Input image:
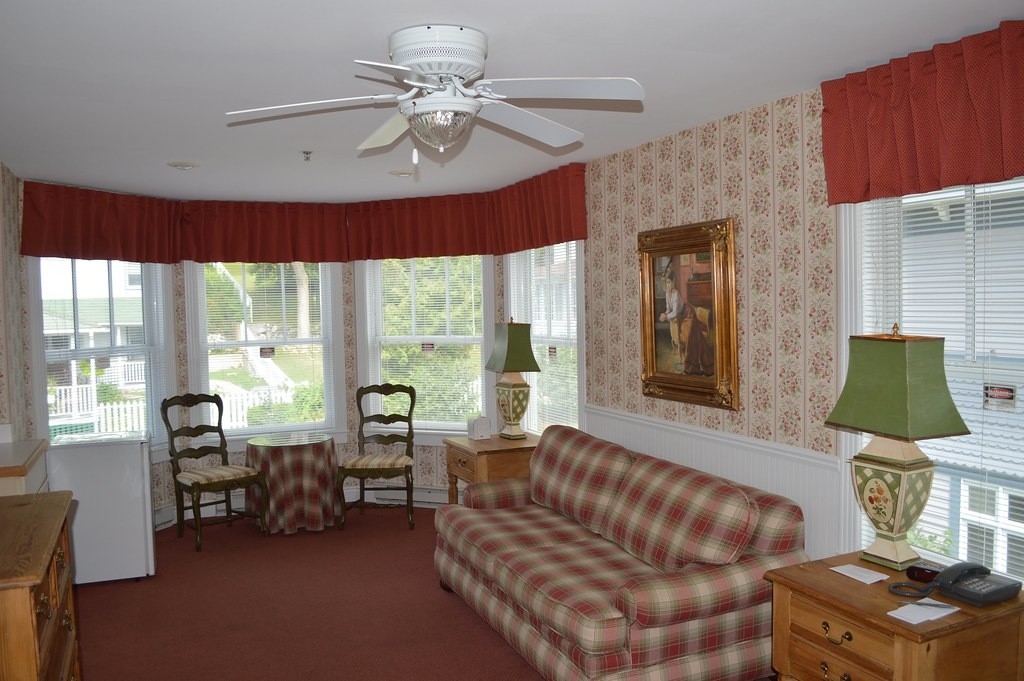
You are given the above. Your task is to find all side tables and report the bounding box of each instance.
[762,549,1024,681]
[443,431,541,506]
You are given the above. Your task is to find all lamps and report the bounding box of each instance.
[399,95,484,151]
[825,321,960,568]
[484,317,541,441]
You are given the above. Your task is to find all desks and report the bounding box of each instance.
[243,432,344,535]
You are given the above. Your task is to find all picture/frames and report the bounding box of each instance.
[634,218,738,410]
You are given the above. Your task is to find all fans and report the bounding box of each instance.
[220,25,647,165]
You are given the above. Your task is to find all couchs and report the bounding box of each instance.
[434,423,803,681]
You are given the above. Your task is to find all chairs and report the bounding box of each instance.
[335,384,419,531]
[159,393,268,552]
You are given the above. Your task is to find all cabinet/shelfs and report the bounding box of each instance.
[47,433,156,585]
[0,489,87,681]
[0,439,50,498]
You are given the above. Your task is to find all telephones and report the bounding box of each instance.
[932,561,1021,607]
